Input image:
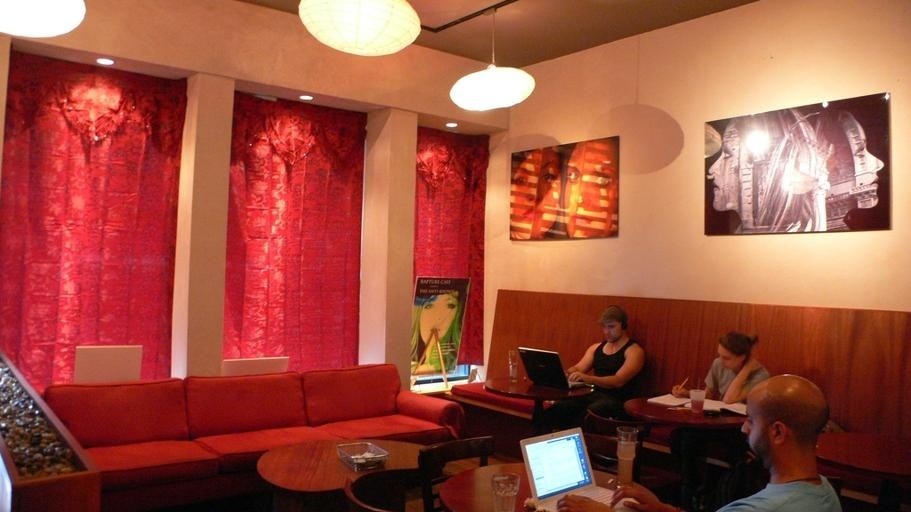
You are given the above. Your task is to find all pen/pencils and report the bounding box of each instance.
[678,376,689,390]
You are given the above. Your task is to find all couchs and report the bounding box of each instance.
[36,362,468,512]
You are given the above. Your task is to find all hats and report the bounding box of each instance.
[596,307,621,323]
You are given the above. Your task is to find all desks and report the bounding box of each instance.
[482,374,595,437]
[622,394,748,512]
[812,431,909,512]
[438,462,662,512]
[253,437,432,511]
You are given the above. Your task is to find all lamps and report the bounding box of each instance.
[449,6,536,113]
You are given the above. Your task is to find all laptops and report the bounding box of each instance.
[520,427,640,512]
[518,347,592,391]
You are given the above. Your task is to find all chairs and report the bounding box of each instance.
[345,468,435,511]
[416,433,495,512]
[581,406,650,485]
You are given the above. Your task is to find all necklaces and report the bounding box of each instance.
[781,475,820,483]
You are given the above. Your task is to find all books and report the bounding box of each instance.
[646,393,691,407]
[684,397,748,416]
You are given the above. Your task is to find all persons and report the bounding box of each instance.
[671,330,770,507]
[510,146,560,239]
[556,372,845,512]
[412,282,462,374]
[805,106,887,230]
[545,304,646,428]
[560,138,619,239]
[708,109,828,233]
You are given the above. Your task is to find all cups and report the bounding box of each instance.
[506,350,519,384]
[491,471,520,512]
[689,389,706,415]
[614,424,639,486]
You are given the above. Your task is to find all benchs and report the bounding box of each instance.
[446,288,911,491]
